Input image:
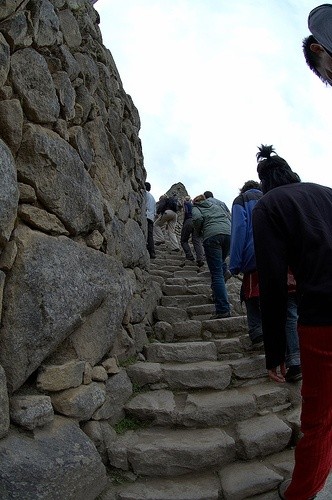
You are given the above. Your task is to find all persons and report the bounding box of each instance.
[173,196,192,212]
[144,182,157,259]
[252,144,332,500]
[229,180,263,347]
[153,195,180,252]
[180,196,205,268]
[302,4,332,87]
[204,191,232,222]
[192,195,232,319]
[248,265,302,383]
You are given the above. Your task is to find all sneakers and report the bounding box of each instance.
[285,367,302,384]
[251,337,264,349]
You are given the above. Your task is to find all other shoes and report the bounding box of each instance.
[210,312,228,319]
[279,478,292,499]
[224,270,231,283]
